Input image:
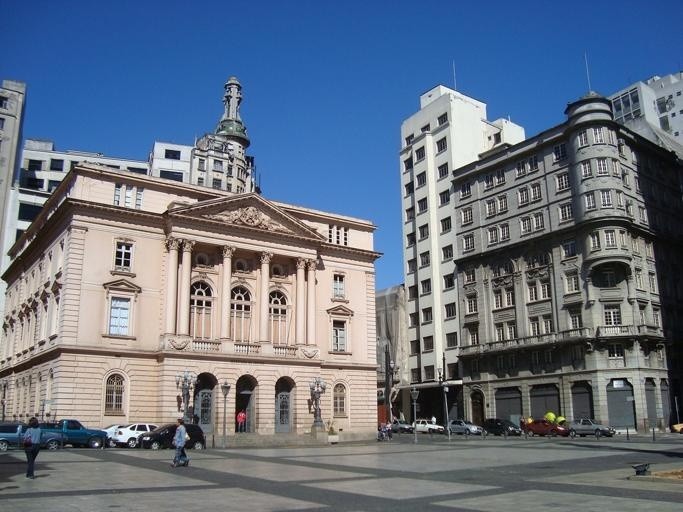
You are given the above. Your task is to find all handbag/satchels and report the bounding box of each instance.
[185,432,190,441]
[22,428,32,448]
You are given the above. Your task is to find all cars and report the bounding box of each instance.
[410,418,444,435]
[482,416,521,436]
[444,418,485,436]
[387,418,413,434]
[518,417,570,438]
[0,416,208,452]
[566,418,617,439]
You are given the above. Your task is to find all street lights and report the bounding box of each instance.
[307,375,327,433]
[437,366,451,434]
[407,383,421,444]
[385,359,395,427]
[173,368,201,423]
[219,381,233,451]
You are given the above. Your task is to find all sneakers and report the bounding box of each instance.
[170,460,192,468]
[26,474,34,480]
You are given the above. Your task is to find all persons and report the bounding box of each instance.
[549,418,556,436]
[171,420,192,467]
[431,416,436,424]
[519,417,526,436]
[23,417,41,478]
[378,418,392,441]
[237,409,246,432]
[527,415,533,423]
